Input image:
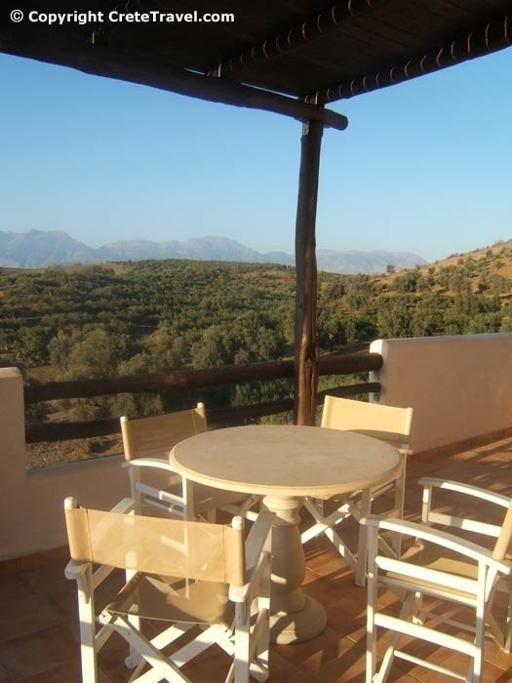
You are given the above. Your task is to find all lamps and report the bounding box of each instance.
[63,495,277,683]
[297,393,414,587]
[119,401,262,601]
[357,475,512,683]
